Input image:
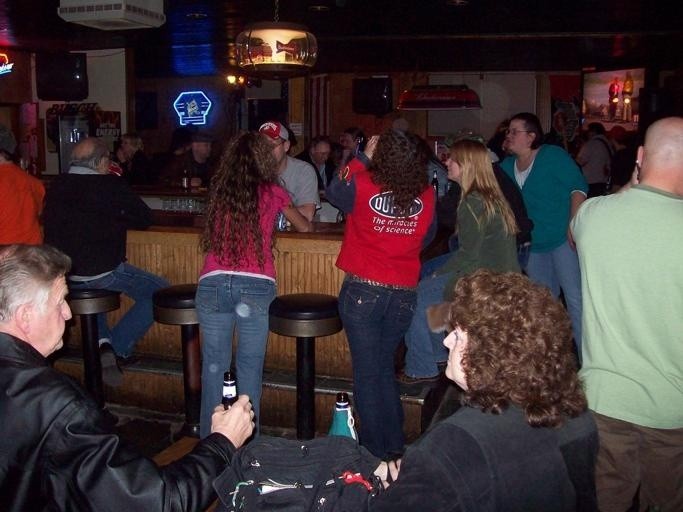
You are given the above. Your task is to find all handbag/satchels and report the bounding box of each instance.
[212,436,391,511]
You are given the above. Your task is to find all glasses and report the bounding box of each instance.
[505,129,532,135]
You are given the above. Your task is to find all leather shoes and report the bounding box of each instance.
[100,342,122,387]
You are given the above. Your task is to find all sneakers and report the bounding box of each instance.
[397,371,439,384]
[400,361,446,369]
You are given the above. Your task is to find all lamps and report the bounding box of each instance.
[235,0,319,81]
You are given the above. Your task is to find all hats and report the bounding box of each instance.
[259,121,288,139]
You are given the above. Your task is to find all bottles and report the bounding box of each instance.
[278,210,287,231]
[329,392,359,444]
[182,169,189,188]
[430,170,439,201]
[222,371,238,409]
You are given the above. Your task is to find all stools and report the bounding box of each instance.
[272,302,340,443]
[154,286,207,436]
[68,289,121,425]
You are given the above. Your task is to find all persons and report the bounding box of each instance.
[117,116,640,222]
[572,116,682,511]
[373,268,598,511]
[0,244,255,511]
[45,135,171,383]
[326,130,436,471]
[397,134,524,385]
[2,135,44,247]
[499,114,586,370]
[196,129,311,443]
